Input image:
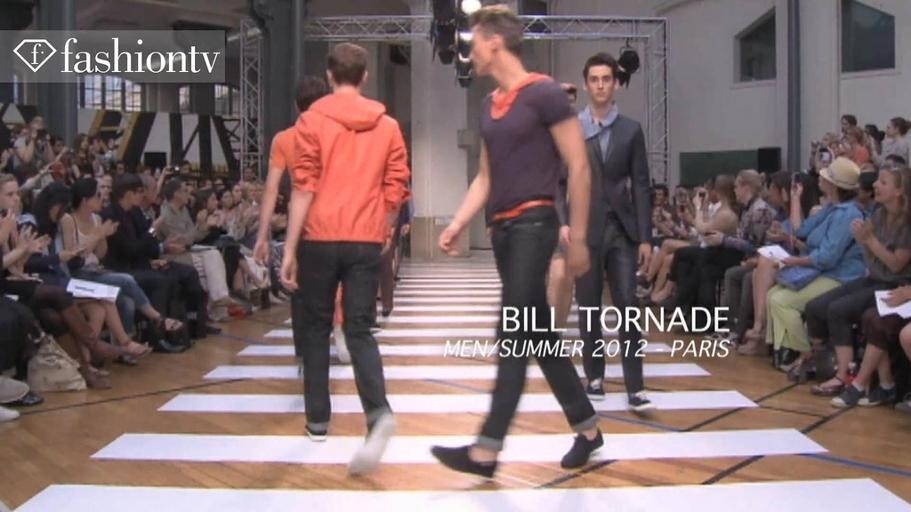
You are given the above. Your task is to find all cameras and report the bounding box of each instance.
[170,165,180,174]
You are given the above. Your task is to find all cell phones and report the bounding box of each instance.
[699,192,705,197]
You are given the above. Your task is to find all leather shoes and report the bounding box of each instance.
[0,405,19,422]
[561,427,604,468]
[431,445,495,478]
[11,393,42,406]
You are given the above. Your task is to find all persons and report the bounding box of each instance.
[280,42,412,475]
[576,53,651,413]
[2,116,289,430]
[545,82,578,333]
[252,75,327,370]
[371,195,411,331]
[430,4,605,479]
[637,114,911,410]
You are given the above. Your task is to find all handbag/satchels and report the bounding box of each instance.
[27,349,87,392]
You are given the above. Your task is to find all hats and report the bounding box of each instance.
[819,156,863,191]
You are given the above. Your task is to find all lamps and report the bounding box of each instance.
[612,38,644,90]
[431,0,484,87]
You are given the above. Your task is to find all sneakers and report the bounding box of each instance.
[304,423,328,439]
[112,313,221,365]
[586,382,605,400]
[628,390,650,410]
[708,329,897,408]
[349,410,395,474]
